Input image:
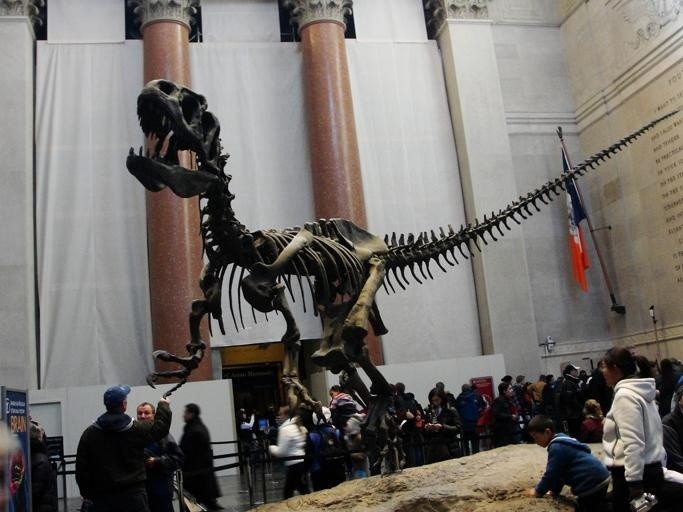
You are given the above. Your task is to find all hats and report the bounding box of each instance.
[565,364,581,374]
[103,384,131,406]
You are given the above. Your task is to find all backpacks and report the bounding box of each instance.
[314,427,349,471]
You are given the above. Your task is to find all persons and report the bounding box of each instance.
[176,402,224,512]
[523,414,612,511]
[73,384,171,511]
[601,345,669,511]
[134,402,184,511]
[29,419,57,511]
[235,356,682,502]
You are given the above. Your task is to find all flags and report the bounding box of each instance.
[561,148,592,293]
